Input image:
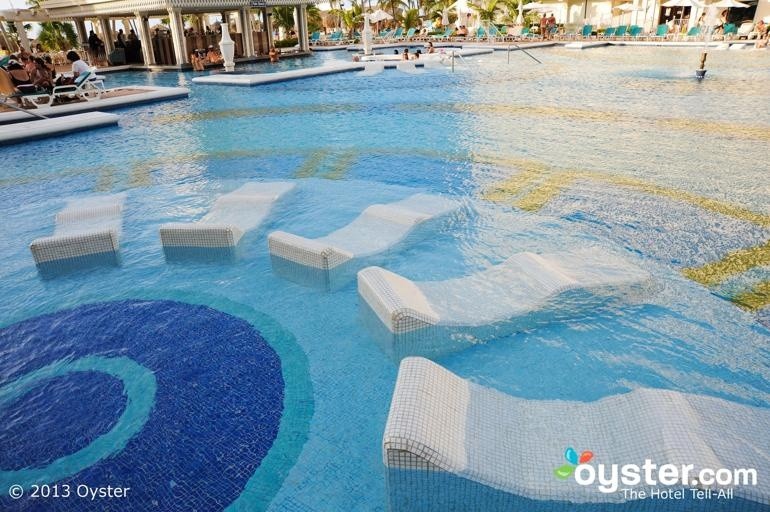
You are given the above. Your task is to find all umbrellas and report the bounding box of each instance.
[704,1,750,26]
[614,3,641,25]
[662,1,707,33]
[518,0,551,33]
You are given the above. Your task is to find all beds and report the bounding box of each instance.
[28,177,769,512]
[359,57,446,74]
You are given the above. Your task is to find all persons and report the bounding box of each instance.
[746,20,766,40]
[715,10,730,33]
[7,25,223,107]
[307,12,557,62]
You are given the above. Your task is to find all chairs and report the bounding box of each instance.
[304,13,770,45]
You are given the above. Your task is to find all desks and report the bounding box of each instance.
[0,10,112,113]
